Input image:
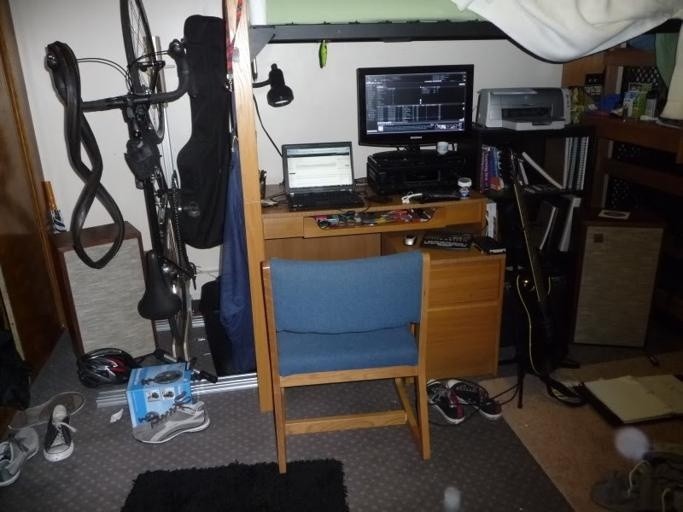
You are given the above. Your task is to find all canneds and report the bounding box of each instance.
[319,214,355,229]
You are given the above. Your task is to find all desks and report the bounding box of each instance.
[261,181,507,382]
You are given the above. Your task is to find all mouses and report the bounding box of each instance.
[404,234,416,246]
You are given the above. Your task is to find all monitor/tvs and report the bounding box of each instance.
[357,64,474,157]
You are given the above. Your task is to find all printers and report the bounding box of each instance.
[476,87,572,132]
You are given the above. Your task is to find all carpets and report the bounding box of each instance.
[121,458,352,512]
[475,351,683,511]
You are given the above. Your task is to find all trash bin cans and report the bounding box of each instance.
[197,279,257,378]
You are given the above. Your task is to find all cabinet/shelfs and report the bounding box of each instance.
[470,121,595,367]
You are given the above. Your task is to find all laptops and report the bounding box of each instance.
[281,142,364,212]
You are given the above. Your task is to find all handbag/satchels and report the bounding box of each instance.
[0,329,30,411]
[590,451,683,511]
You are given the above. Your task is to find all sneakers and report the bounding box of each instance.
[132,401,210,444]
[445,379,503,421]
[0,426,39,487]
[43,404,75,463]
[426,378,465,424]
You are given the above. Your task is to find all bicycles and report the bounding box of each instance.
[45,1,219,385]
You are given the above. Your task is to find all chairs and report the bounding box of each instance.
[261,250,432,474]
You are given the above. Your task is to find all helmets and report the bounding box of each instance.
[76,348,137,388]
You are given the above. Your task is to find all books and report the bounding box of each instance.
[480,137,591,252]
[573,370,682,431]
